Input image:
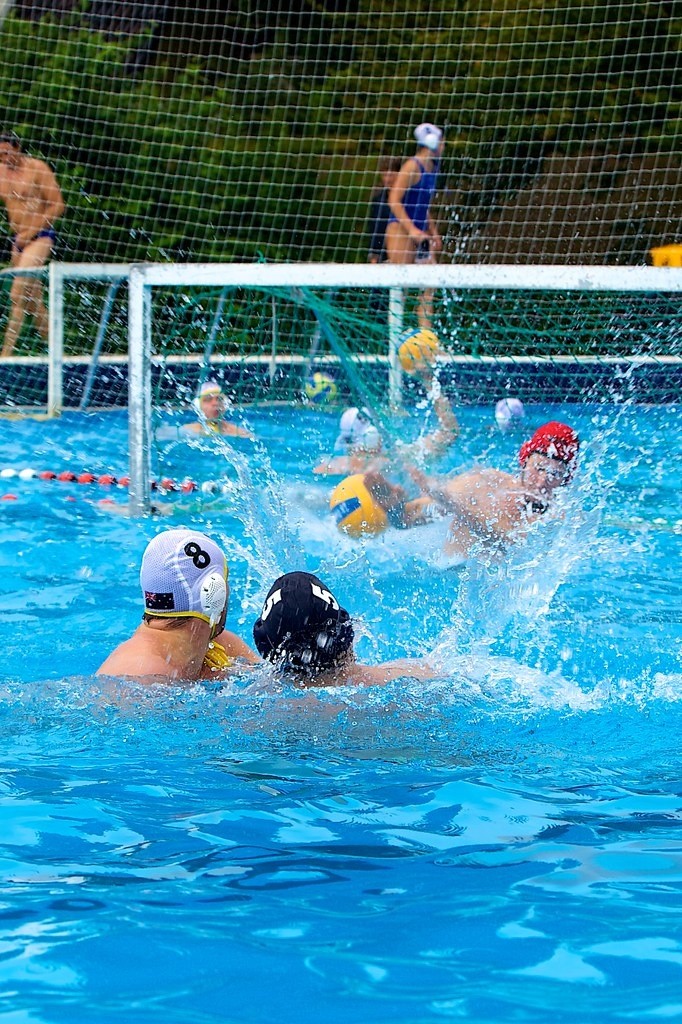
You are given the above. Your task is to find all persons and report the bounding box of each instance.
[72,529,268,720]
[364,422,582,559]
[235,570,445,736]
[0,125,63,359]
[179,120,460,474]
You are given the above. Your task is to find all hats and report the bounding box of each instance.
[329,473,390,539]
[140,530,227,628]
[413,122,442,152]
[519,421,579,485]
[253,572,355,678]
[495,398,522,435]
[340,406,373,444]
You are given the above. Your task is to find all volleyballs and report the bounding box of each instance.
[495,398,524,429]
[397,328,440,371]
[306,372,337,404]
[330,474,391,540]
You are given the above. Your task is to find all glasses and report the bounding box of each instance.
[418,239,430,259]
[198,394,225,403]
[526,460,570,481]
[366,482,391,497]
